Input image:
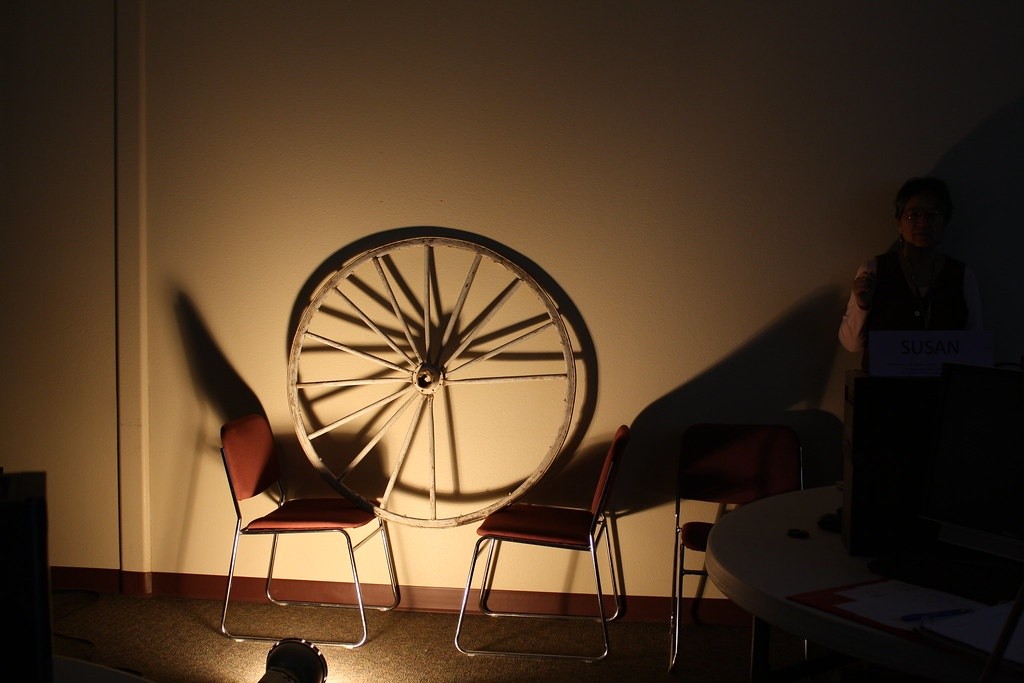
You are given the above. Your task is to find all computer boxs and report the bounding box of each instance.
[841,368,942,558]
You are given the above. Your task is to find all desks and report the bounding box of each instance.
[704,487,1024,683]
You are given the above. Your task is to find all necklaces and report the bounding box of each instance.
[900,242,936,321]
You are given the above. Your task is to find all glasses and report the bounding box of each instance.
[902,207,947,226]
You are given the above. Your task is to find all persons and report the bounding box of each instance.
[839,174,984,374]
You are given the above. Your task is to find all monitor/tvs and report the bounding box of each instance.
[918,360,1024,565]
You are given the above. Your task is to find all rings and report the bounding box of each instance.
[856,277,860,280]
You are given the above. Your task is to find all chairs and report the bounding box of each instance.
[666,424,810,676]
[219,416,401,648]
[454,424,630,664]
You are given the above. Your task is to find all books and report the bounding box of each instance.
[785,577,1024,670]
